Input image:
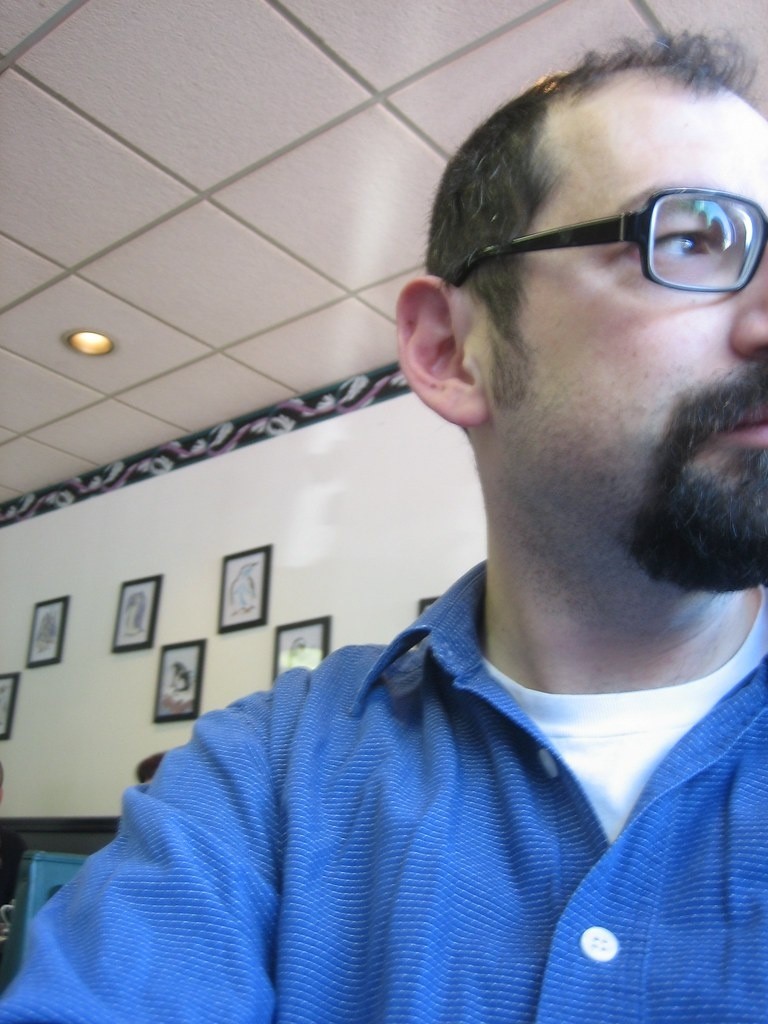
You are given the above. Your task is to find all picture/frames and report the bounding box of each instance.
[0,673,19,741]
[26,596,69,668]
[272,616,330,681]
[111,574,162,654]
[216,544,270,634]
[152,639,205,724]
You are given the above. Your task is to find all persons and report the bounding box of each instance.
[0,30,768,1022]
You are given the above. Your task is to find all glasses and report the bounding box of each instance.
[453,187,768,288]
